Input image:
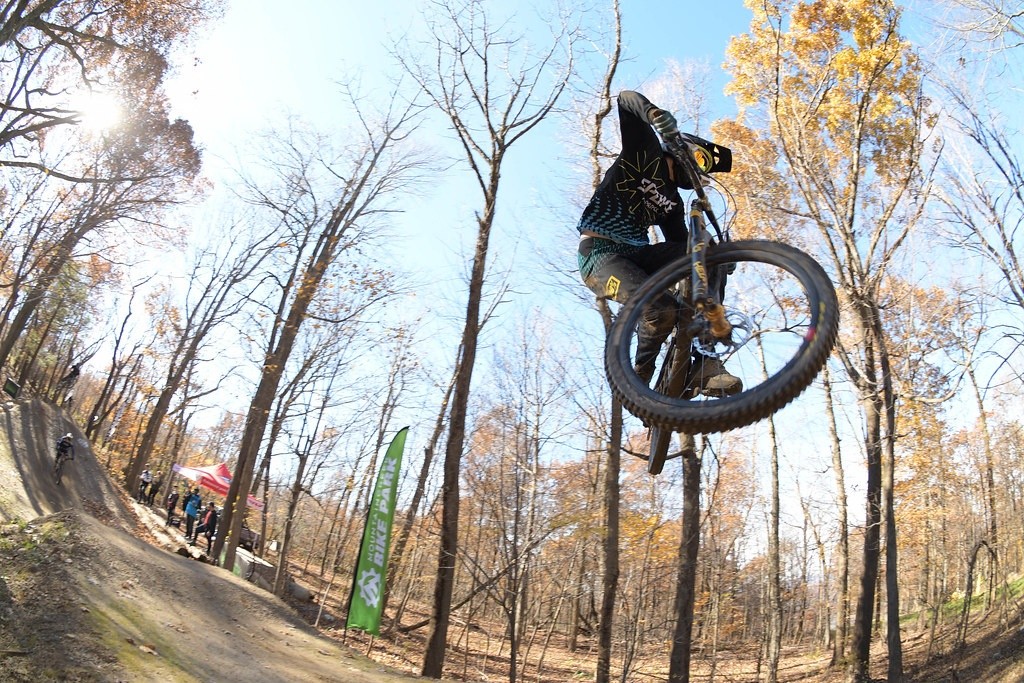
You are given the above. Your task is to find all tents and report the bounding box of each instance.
[161,462,265,551]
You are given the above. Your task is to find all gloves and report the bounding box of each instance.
[727,262,736,273]
[648,106,679,143]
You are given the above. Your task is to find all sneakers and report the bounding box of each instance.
[691,357,743,396]
[633,363,656,386]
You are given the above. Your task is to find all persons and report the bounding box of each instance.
[138,463,163,506]
[166,485,179,527]
[182,483,210,539]
[577,89,742,397]
[57,432,75,464]
[190,502,217,555]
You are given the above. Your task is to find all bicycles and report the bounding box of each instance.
[54,448,73,486]
[602,108,840,475]
[61,369,79,389]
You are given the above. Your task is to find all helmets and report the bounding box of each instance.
[660,132,732,189]
[67,432,74,439]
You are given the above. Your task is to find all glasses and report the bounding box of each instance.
[689,144,712,173]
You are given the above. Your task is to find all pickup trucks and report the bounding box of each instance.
[200,508,261,552]
